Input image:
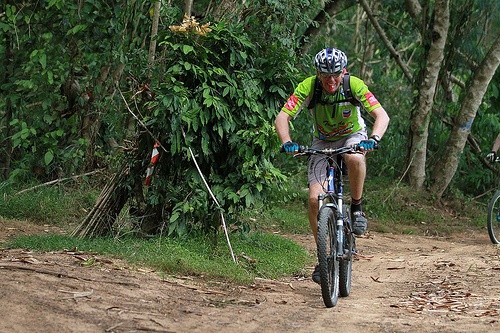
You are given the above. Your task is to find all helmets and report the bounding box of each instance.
[313,47,348,73]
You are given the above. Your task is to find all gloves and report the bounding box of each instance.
[487,151,496,163]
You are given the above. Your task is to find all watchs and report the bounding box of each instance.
[370,134,382,143]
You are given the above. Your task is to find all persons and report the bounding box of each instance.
[275,48,391,285]
[486,132,500,164]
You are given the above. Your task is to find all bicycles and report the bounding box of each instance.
[479,148,500,245]
[279,140,379,307]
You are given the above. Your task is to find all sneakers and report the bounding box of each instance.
[312,264,321,284]
[351,210,367,235]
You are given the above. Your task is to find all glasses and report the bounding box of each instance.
[320,70,343,78]
